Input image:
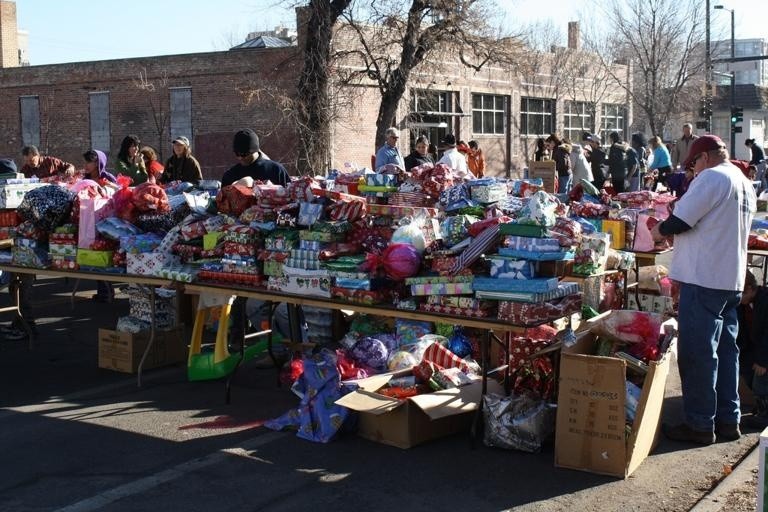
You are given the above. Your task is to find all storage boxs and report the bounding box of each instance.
[553,310,678,480]
[527,161,559,195]
[98,326,181,373]
[334,367,505,450]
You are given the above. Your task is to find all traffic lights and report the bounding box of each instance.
[695,121,706,129]
[704,96,713,116]
[697,96,706,118]
[734,105,743,122]
[729,103,737,126]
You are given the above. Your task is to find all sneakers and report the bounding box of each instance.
[715,424,741,440]
[661,424,716,445]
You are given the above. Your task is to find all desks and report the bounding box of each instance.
[620,248,673,310]
[663,171,686,196]
[184,280,558,456]
[747,250,768,287]
[0,265,182,388]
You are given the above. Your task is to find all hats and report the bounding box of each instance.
[172,136,189,146]
[587,134,601,141]
[233,128,259,153]
[681,135,726,166]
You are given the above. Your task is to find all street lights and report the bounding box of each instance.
[712,4,737,163]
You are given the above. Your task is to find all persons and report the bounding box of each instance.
[245,299,304,368]
[113,135,148,185]
[222,129,291,187]
[745,139,768,196]
[21,145,75,178]
[141,146,164,183]
[160,136,203,185]
[649,135,757,443]
[737,270,768,416]
[536,122,700,196]
[374,128,485,179]
[83,149,116,183]
